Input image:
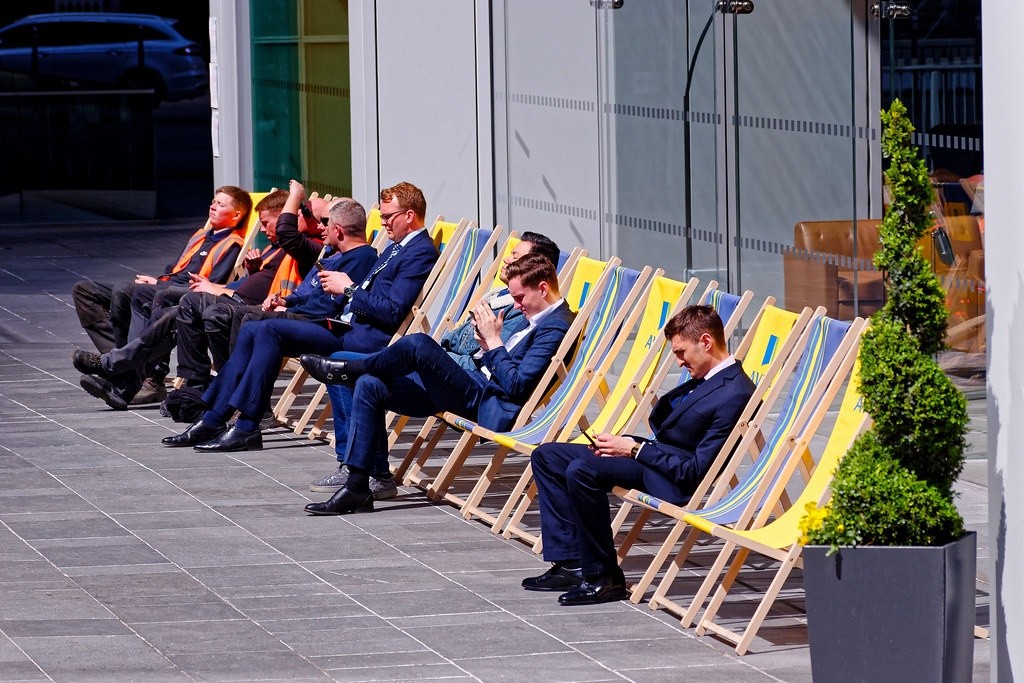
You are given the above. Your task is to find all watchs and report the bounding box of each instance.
[344,284,357,297]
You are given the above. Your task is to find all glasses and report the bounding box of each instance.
[299,202,320,224]
[380,208,416,220]
[319,217,329,227]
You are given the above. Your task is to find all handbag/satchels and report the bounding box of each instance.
[165,386,205,422]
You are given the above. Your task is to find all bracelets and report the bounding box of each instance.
[630,443,641,458]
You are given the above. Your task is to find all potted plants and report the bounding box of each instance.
[799,98,977,683]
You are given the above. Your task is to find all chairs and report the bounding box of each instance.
[206,188,989,656]
[959,174,985,214]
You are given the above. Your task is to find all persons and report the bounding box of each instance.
[310,231,561,497]
[71,185,251,354]
[162,182,442,453]
[521,305,763,605]
[229,201,380,428]
[127,189,290,401]
[160,198,353,416]
[299,254,583,514]
[72,179,329,410]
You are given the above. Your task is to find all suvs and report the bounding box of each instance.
[0,13,209,112]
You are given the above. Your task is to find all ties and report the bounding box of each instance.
[365,244,402,283]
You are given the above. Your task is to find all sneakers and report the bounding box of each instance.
[368,476,400,499]
[71,349,112,378]
[160,377,201,416]
[128,377,167,404]
[309,465,351,492]
[80,374,127,411]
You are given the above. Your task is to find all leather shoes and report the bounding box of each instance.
[300,353,356,389]
[194,426,262,452]
[523,561,585,590]
[558,567,626,605]
[161,417,229,446]
[304,486,373,514]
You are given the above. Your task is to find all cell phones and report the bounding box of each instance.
[468,311,476,322]
[579,428,599,450]
[314,261,324,272]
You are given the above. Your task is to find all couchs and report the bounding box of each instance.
[784,216,988,320]
[941,250,986,353]
